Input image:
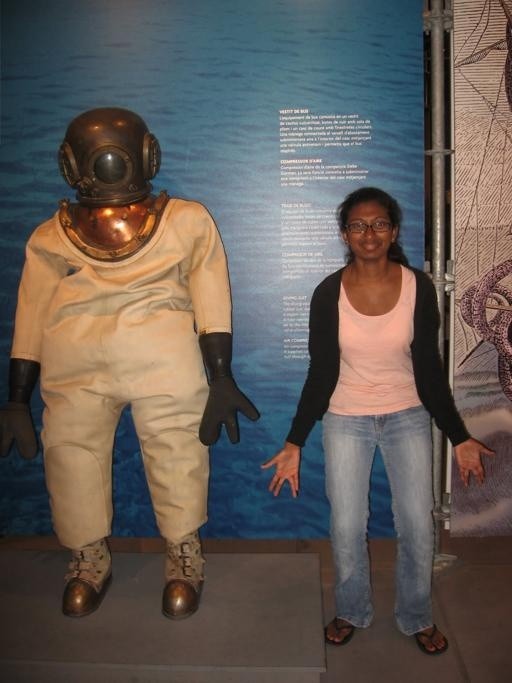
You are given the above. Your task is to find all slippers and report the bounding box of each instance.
[325,616,356,647]
[413,624,448,656]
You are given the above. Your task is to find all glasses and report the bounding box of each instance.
[345,220,394,233]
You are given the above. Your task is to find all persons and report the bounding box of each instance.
[259,187,496,653]
[1,102,264,616]
